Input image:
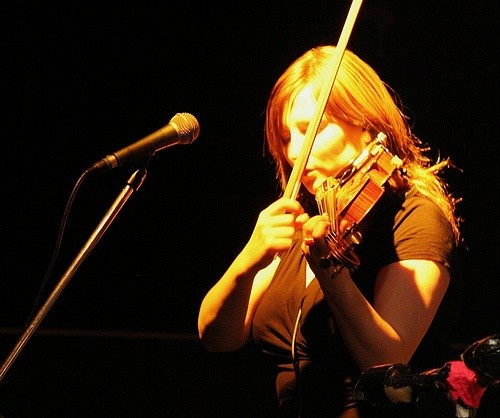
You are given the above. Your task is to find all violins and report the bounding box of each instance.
[313,136,412,279]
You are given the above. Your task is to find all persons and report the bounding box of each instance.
[197,45,464,418]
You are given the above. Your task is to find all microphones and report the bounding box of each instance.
[95,112,200,171]
